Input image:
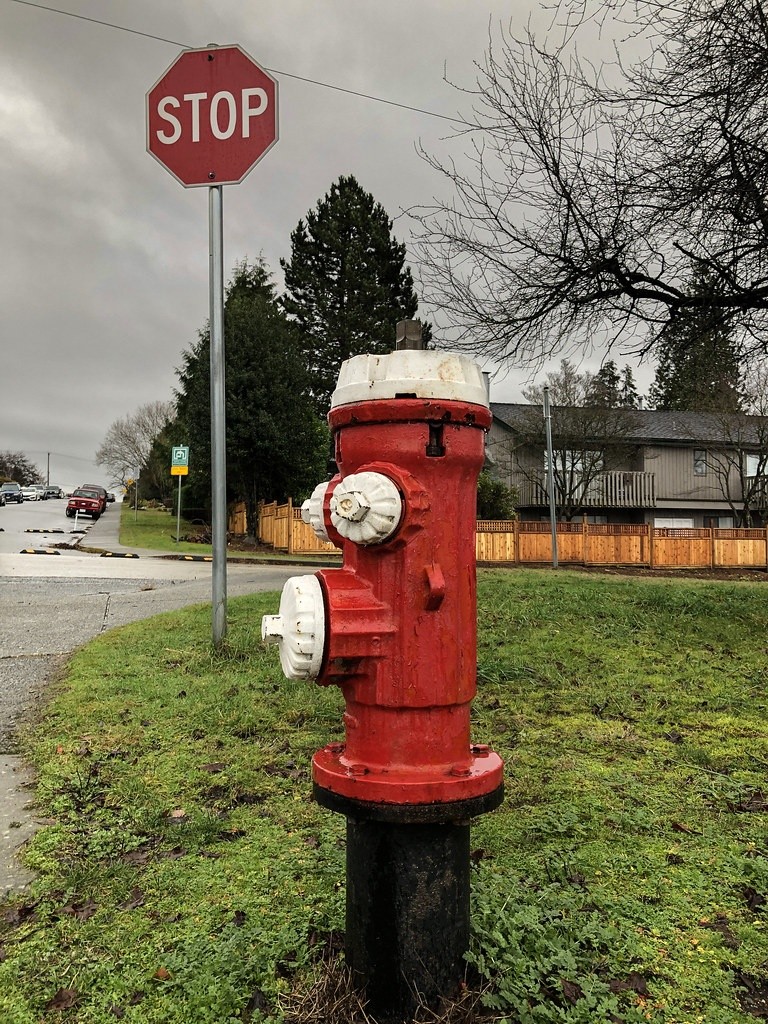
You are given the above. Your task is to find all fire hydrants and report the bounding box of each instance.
[262,350,506,1024]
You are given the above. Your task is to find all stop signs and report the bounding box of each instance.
[146,43,278,188]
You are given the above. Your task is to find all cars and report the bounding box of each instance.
[0,488,6,506]
[44,486,65,499]
[20,486,41,502]
[107,493,115,502]
[29,484,47,500]
[66,492,74,497]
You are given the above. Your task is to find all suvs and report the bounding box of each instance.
[2,482,24,504]
[66,487,105,520]
[77,484,108,514]
